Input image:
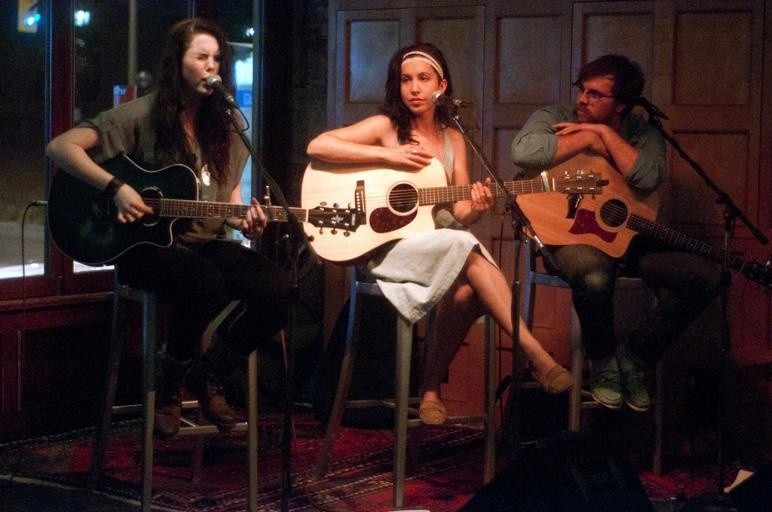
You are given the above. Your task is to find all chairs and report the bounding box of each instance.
[313,257,500,510]
[89,246,259,512]
[511,238,670,479]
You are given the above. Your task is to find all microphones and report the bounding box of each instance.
[632,94,672,121]
[430,91,475,110]
[207,74,240,112]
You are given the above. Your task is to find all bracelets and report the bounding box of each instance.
[104,176,126,197]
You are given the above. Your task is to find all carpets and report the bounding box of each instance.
[1,399,751,512]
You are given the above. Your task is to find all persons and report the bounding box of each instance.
[308,43,574,425]
[511,54,723,412]
[46,18,268,437]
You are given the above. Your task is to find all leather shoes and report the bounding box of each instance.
[188,371,236,430]
[144,375,185,440]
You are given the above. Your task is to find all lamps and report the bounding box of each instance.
[19,4,42,33]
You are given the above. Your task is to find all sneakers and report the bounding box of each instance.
[589,359,623,411]
[622,368,653,413]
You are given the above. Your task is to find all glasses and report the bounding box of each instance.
[576,83,614,102]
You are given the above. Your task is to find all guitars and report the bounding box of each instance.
[300,155,609,263]
[46,145,361,265]
[511,152,772,292]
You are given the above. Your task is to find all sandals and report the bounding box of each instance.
[418,384,447,424]
[527,359,575,395]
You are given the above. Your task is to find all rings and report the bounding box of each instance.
[256,222,259,225]
[413,149,416,154]
